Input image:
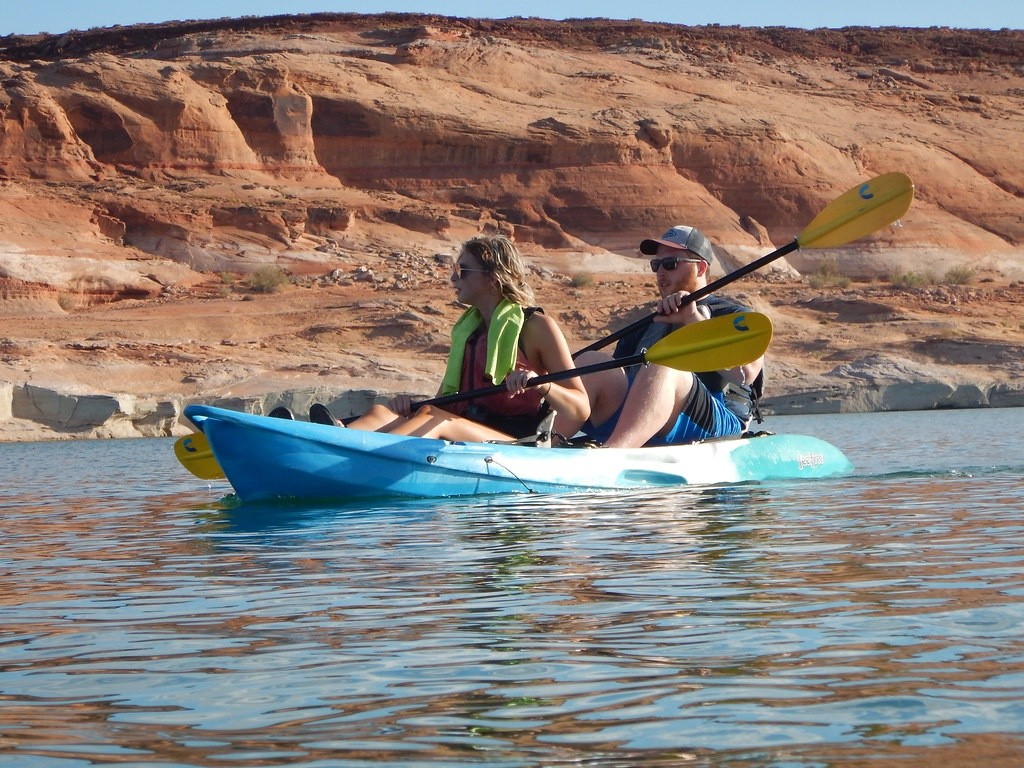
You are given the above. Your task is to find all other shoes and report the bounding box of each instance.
[309,403,347,428]
[268,406,294,419]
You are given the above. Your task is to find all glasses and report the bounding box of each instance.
[650,257,705,272]
[452,263,495,278]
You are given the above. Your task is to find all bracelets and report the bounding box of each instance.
[538,382,551,395]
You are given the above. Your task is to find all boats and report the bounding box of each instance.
[183,403,855,498]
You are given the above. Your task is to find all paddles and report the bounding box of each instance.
[572,170,914,364]
[171,310,774,480]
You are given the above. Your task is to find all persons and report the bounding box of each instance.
[269,237,591,448]
[552,225,765,449]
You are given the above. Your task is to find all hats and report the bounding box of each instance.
[640,225,712,266]
